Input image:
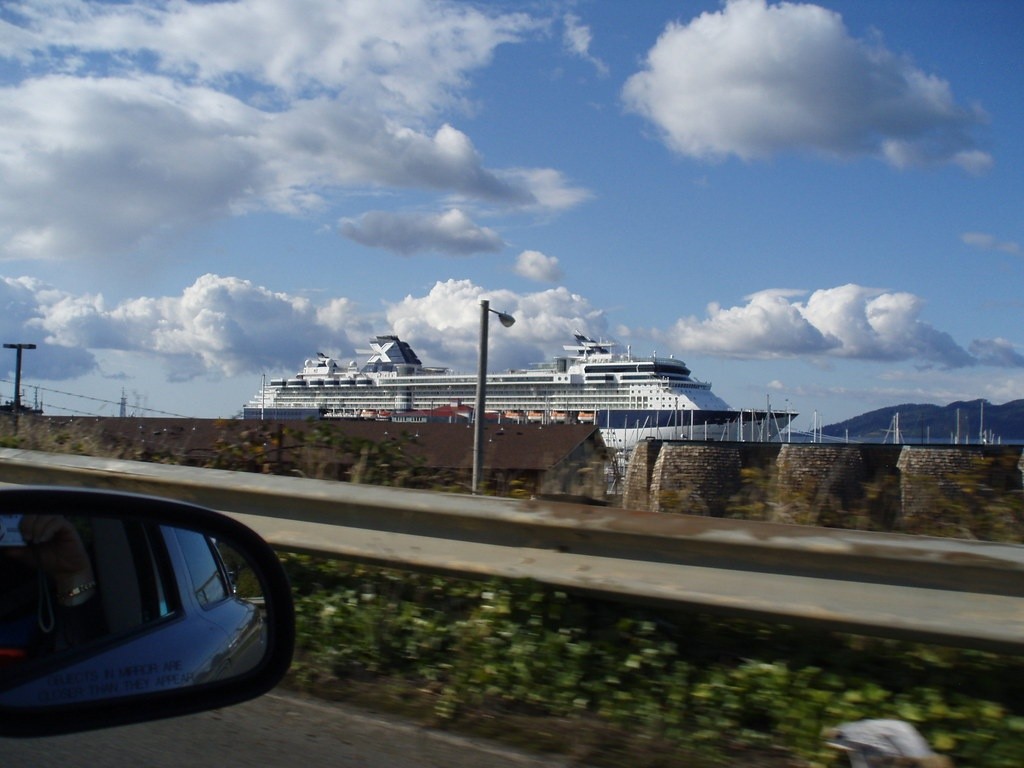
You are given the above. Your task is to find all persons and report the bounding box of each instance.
[4,514,110,660]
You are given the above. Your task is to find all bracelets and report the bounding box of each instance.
[56,581,96,601]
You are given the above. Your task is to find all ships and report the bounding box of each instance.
[242,330,800,474]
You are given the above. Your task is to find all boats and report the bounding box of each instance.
[377,410,392,419]
[504,411,525,421]
[578,411,595,424]
[527,411,543,423]
[360,410,376,420]
[549,412,567,422]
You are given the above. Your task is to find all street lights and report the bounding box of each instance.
[2,342,36,435]
[468,297,517,496]
